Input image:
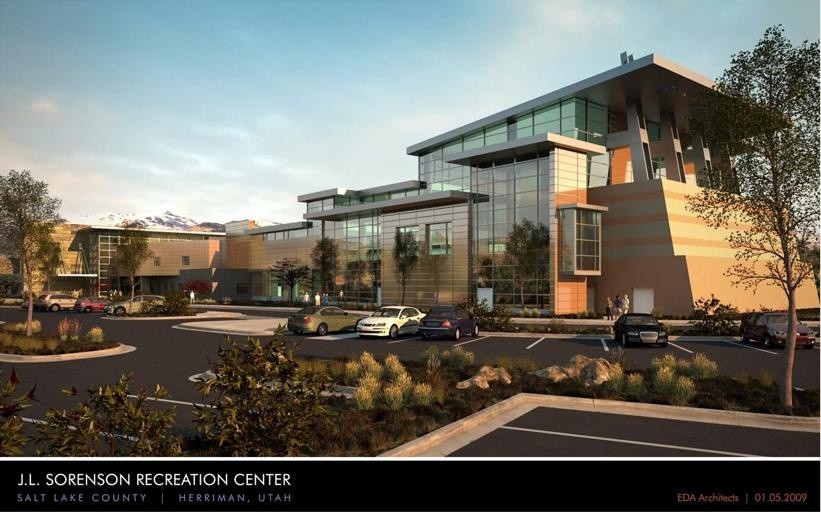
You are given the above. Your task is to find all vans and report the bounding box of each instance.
[36,293,79,313]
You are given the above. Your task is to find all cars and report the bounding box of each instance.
[287,305,368,337]
[355,305,424,340]
[739,310,816,351]
[613,312,669,350]
[76,296,108,313]
[418,305,480,343]
[105,292,168,318]
